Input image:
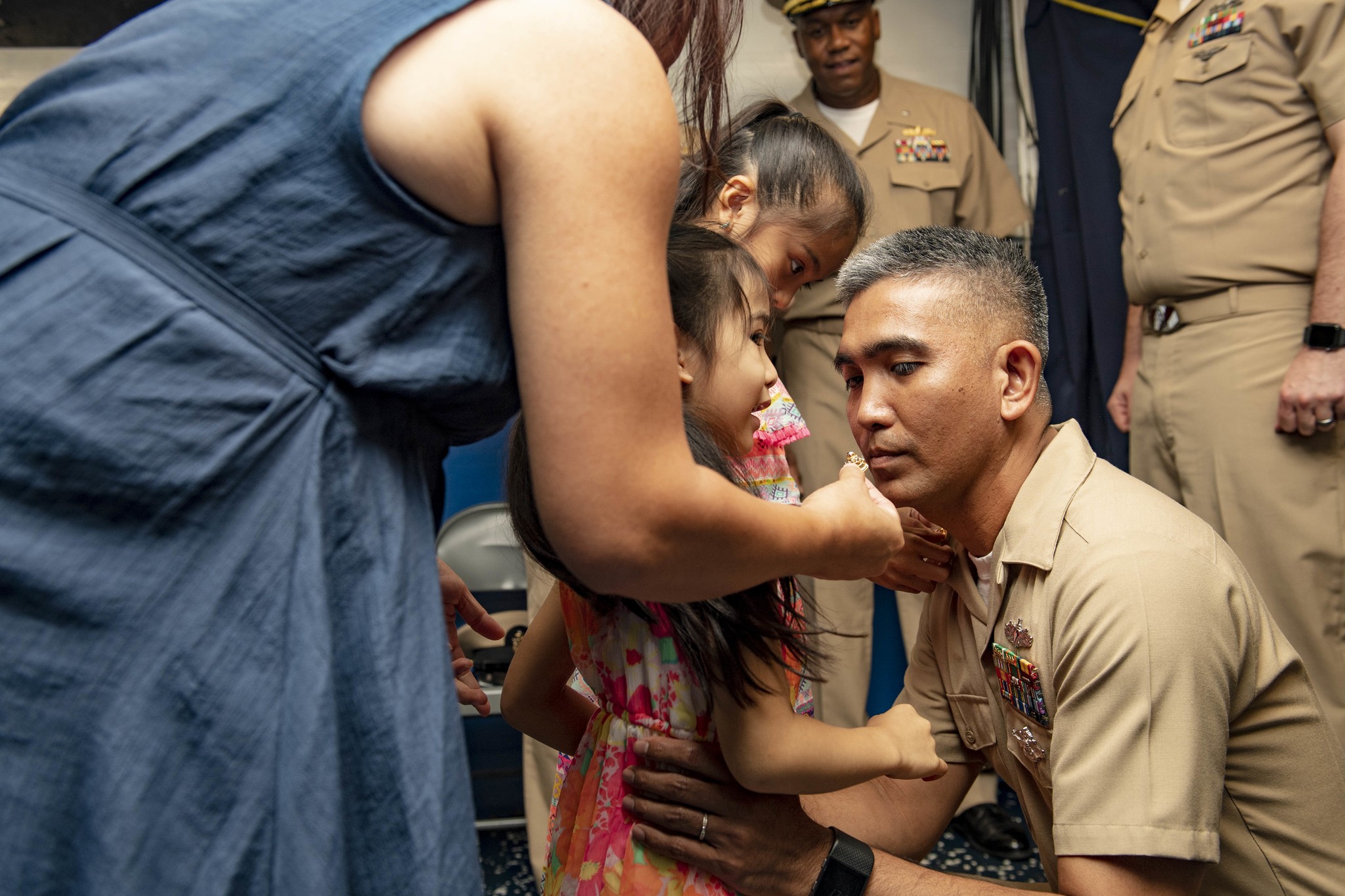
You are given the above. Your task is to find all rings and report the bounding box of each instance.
[699,813,709,841]
[1317,416,1333,427]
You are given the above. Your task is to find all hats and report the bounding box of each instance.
[767,0,875,19]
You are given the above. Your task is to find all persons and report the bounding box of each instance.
[0,0,908,896]
[623,225,1345,896]
[772,0,1032,730]
[526,97,868,887]
[1106,0,1345,767]
[500,228,954,896]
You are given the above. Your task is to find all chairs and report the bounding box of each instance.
[437,503,525,830]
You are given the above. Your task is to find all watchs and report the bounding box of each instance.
[1303,322,1345,352]
[808,825,874,896]
